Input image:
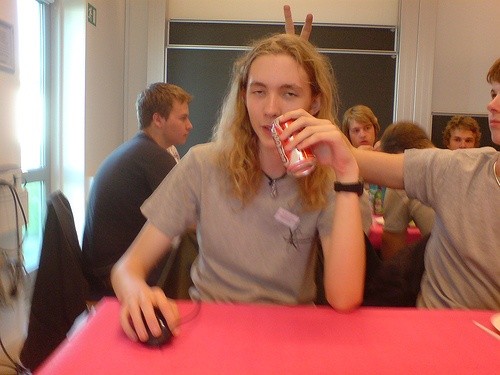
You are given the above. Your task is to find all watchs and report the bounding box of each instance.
[333,176,364,197]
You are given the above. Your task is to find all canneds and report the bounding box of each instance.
[271,118,318,179]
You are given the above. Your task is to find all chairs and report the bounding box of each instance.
[15,191,85,375]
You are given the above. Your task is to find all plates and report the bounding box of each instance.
[492,314,500,331]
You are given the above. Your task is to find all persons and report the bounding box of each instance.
[442,114,481,150]
[110,35,366,343]
[314,104,437,308]
[80,81,192,300]
[284,4,500,312]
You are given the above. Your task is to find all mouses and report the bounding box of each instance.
[143,306,172,347]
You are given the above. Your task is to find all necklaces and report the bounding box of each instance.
[260,169,287,198]
[493,161,500,186]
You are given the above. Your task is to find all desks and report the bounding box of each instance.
[34,297,500,375]
[176,207,421,299]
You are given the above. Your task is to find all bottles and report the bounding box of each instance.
[374,186,384,214]
